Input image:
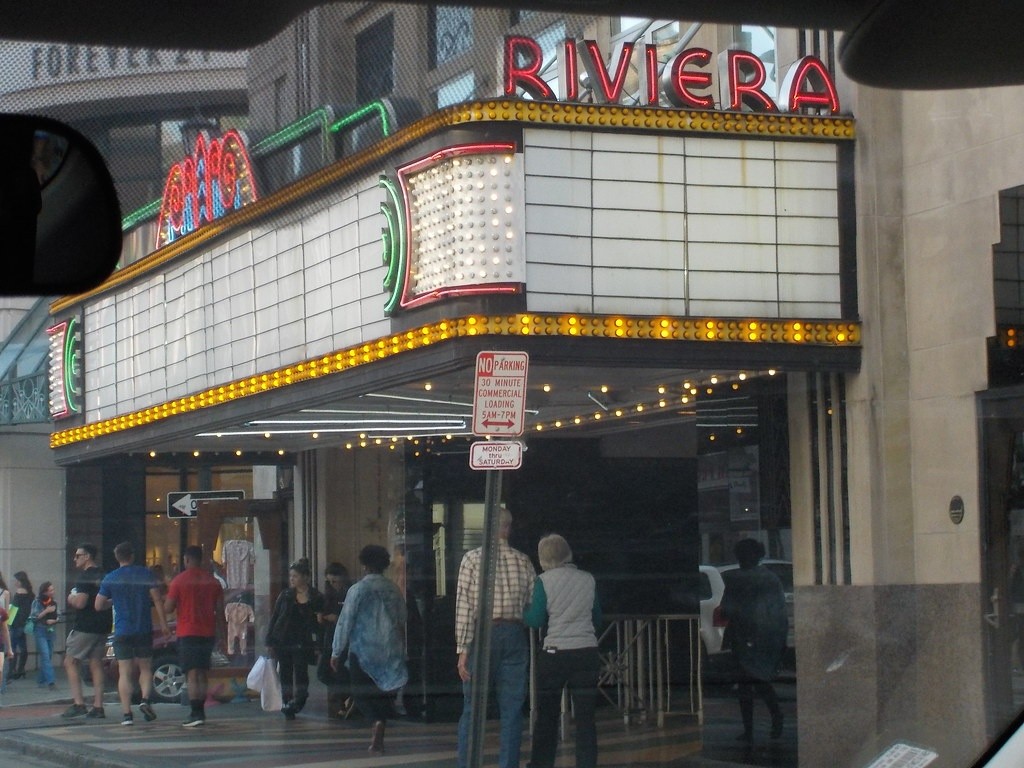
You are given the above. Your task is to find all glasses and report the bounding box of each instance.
[72,553,88,559]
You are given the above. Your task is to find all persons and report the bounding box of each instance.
[8,571,35,679]
[264,558,325,720]
[0,571,10,694]
[60,543,113,719]
[455,508,536,768]
[95,542,171,725]
[720,539,789,744]
[330,546,408,753]
[316,562,355,720]
[31,581,60,690]
[163,545,228,727]
[0,605,14,691]
[523,534,602,768]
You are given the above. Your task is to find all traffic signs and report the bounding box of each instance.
[167,491,245,518]
[472,350,529,437]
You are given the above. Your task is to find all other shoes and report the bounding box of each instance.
[39,682,45,689]
[60,703,88,720]
[86,704,105,718]
[368,719,386,756]
[139,701,157,721]
[180,714,206,727]
[336,695,357,721]
[119,712,134,726]
[47,682,57,692]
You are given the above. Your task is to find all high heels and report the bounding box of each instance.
[18,669,26,678]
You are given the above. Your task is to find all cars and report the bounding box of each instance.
[699,558,793,684]
[77,582,326,702]
